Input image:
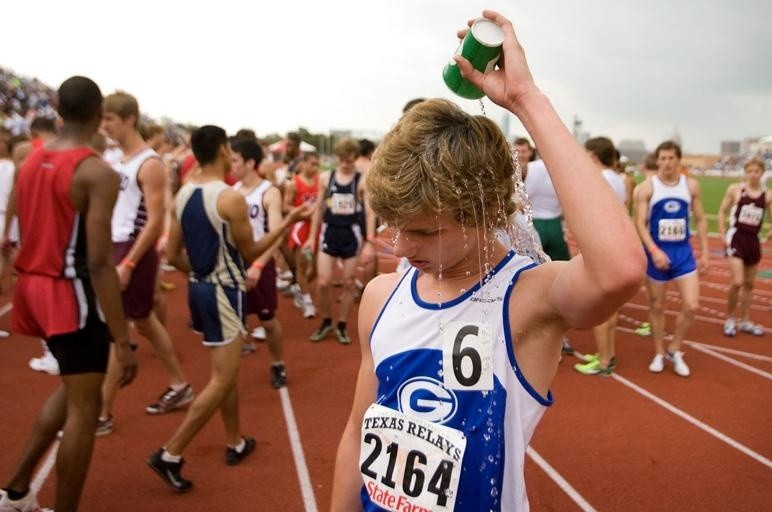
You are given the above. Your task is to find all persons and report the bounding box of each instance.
[632,140,710,378]
[512,137,530,180]
[266,131,300,288]
[522,158,574,355]
[281,151,322,320]
[227,140,288,390]
[574,134,626,376]
[145,125,317,492]
[308,137,377,345]
[146,126,165,151]
[0,115,60,254]
[56,94,194,438]
[0,75,139,511]
[330,9,647,512]
[352,137,375,176]
[640,154,658,179]
[1,128,17,338]
[719,159,768,337]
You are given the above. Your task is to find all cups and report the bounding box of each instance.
[442,19,507,101]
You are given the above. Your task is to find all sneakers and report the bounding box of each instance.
[724,319,737,337]
[275,277,288,291]
[584,351,617,369]
[738,322,764,336]
[145,445,193,492]
[249,326,266,340]
[241,343,259,356]
[29,354,61,376]
[269,361,287,387]
[161,263,176,272]
[634,321,652,336]
[574,361,611,377]
[310,319,332,341]
[278,271,293,279]
[283,284,299,297]
[648,353,665,372]
[160,281,175,291]
[334,322,352,343]
[665,349,691,377]
[0,487,53,512]
[145,384,195,415]
[56,416,116,438]
[302,298,316,319]
[293,293,304,308]
[562,341,576,354]
[225,434,255,466]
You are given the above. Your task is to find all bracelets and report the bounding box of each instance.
[719,226,725,232]
[251,261,264,272]
[120,260,134,271]
[366,235,374,240]
[647,246,658,251]
